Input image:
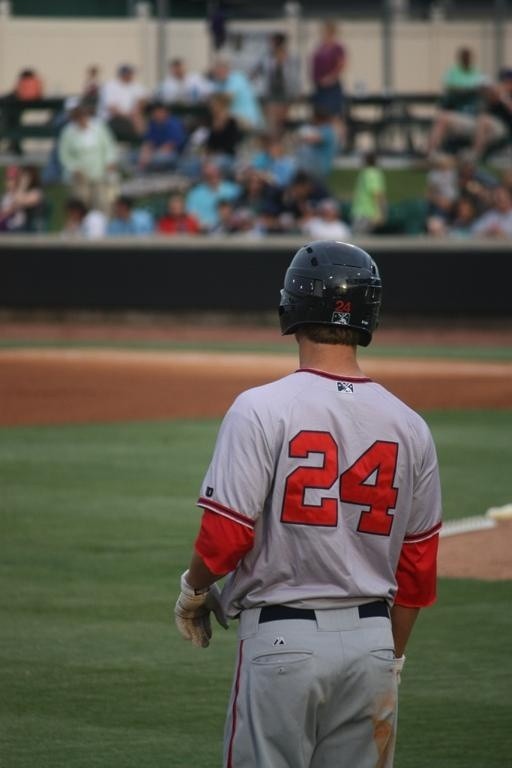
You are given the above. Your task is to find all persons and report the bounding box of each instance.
[173,240,443,767]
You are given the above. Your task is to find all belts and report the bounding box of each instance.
[246,602,395,626]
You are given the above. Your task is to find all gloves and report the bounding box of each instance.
[392,653,407,687]
[174,565,229,647]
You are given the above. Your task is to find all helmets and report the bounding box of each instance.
[274,237,382,338]
